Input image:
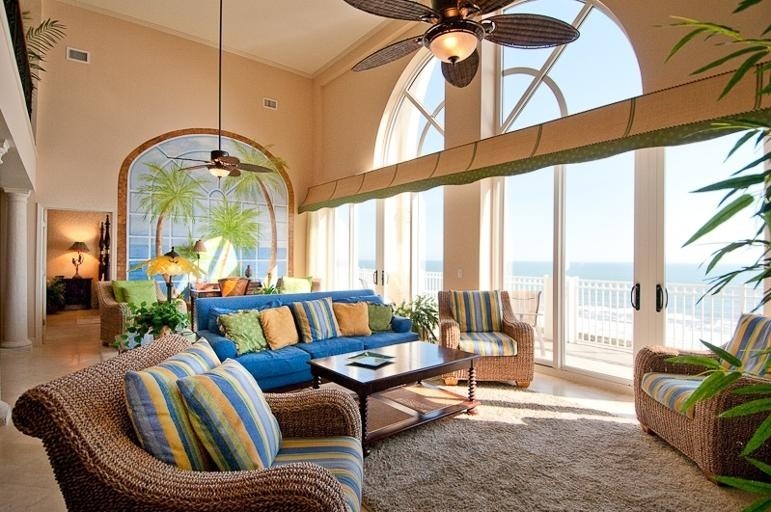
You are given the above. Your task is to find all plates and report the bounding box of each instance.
[348,349,397,368]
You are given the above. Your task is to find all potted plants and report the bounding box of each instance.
[114,300,188,355]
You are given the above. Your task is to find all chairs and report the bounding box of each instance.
[632,309,771,485]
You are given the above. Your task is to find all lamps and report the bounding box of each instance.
[67,241,90,280]
[419,21,487,66]
[129,245,208,303]
[208,165,235,182]
[192,240,207,278]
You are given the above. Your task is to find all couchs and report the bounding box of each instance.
[195,289,419,392]
[97,280,188,347]
[437,289,535,388]
[12,333,363,512]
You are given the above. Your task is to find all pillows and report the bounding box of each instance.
[292,297,342,343]
[258,305,299,351]
[333,295,383,305]
[113,280,158,302]
[123,336,222,472]
[207,299,282,335]
[176,357,283,471]
[332,302,372,337]
[366,302,393,333]
[217,309,267,357]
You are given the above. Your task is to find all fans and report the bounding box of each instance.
[164,2,275,184]
[343,1,582,92]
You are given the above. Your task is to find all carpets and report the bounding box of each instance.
[356,393,771,510]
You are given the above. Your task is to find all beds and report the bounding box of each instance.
[97,214,111,291]
